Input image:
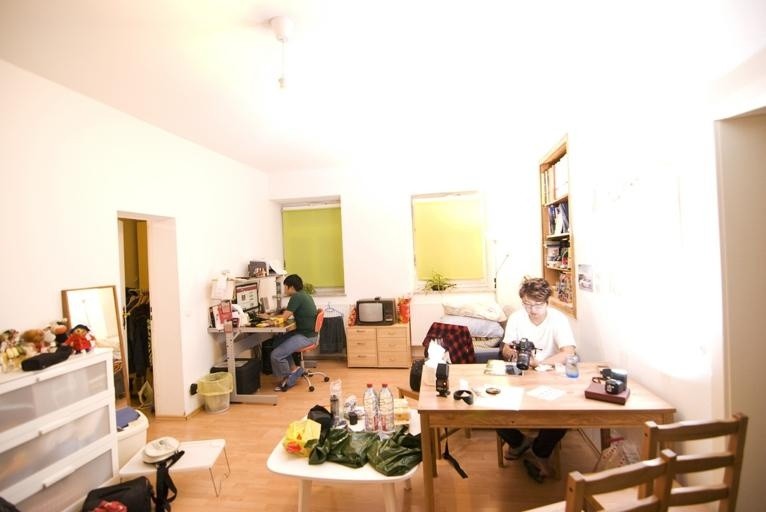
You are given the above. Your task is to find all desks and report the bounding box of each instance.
[417,361,677,512]
[116,438,233,500]
[208,319,297,405]
[267,408,421,512]
[411,293,444,346]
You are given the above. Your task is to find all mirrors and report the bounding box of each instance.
[61,285,131,412]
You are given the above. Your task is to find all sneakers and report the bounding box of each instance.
[274,380,287,391]
[287,367,304,387]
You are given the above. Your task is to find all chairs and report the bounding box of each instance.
[279,310,330,392]
[398,320,477,458]
[497,333,580,468]
[636,411,749,512]
[523,448,677,512]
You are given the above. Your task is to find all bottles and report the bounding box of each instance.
[377,383,395,435]
[565,353,578,378]
[329,395,339,425]
[362,383,378,434]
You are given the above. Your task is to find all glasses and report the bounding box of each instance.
[521,300,545,308]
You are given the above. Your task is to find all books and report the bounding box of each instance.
[584,383,630,406]
[535,149,573,300]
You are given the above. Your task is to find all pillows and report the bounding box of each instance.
[442,314,505,338]
[442,292,508,322]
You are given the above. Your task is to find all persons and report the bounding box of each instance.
[494,275,578,484]
[254,273,320,392]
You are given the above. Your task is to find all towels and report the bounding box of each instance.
[319,317,346,355]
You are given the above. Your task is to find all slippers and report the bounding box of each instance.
[504,437,533,460]
[523,451,547,483]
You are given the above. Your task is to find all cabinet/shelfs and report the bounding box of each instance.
[346,326,378,368]
[377,323,411,369]
[539,136,577,318]
[0,346,120,511]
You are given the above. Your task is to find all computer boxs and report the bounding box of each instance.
[261,339,300,374]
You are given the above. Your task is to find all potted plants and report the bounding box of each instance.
[424,271,456,292]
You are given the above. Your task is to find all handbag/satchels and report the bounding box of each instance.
[80,476,153,512]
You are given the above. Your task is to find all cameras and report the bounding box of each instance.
[513,336,535,370]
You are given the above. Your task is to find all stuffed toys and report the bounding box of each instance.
[0,315,98,376]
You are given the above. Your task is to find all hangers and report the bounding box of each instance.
[126,287,149,315]
[322,304,345,317]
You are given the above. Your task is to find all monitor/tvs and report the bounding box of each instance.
[235,282,259,312]
[356,299,396,326]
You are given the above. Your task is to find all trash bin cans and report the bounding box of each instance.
[199,371,231,414]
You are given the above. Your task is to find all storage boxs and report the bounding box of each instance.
[210,358,261,395]
